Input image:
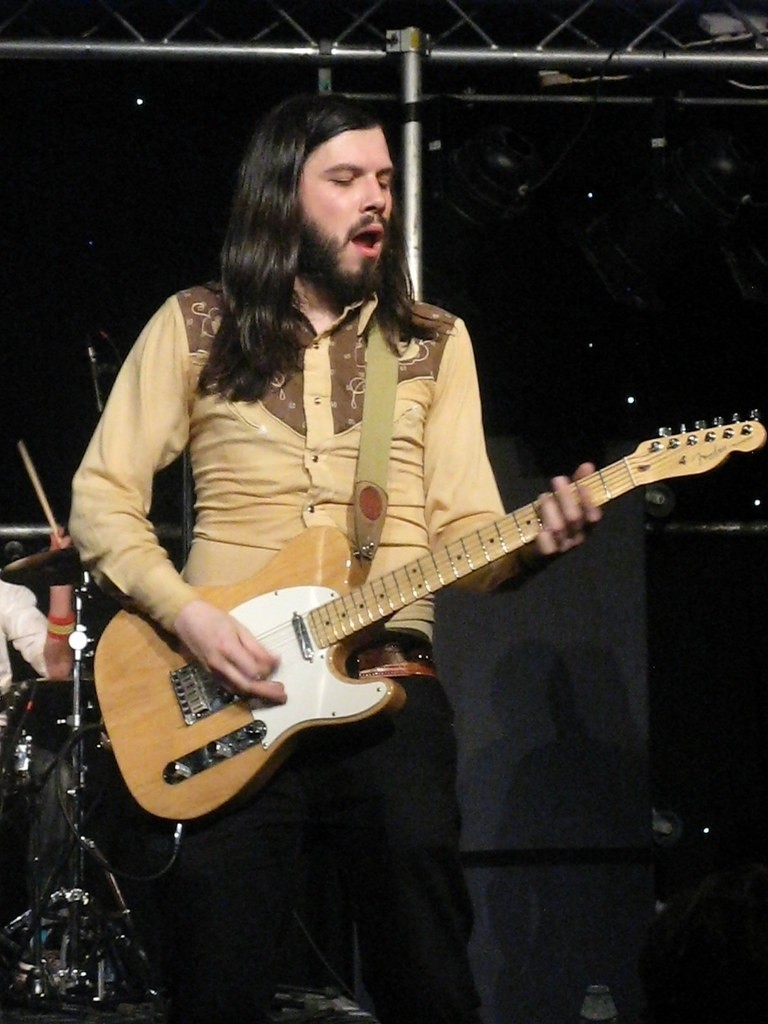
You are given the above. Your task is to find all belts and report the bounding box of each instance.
[358,643,438,678]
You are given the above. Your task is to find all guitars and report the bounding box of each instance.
[92,406,768,825]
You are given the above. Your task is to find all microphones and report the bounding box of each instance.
[87,333,104,414]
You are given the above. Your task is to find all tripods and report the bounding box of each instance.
[0,642,169,1016]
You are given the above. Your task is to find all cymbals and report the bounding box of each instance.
[0,524,193,585]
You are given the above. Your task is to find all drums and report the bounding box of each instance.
[8,673,95,750]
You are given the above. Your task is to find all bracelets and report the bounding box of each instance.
[46,610,76,639]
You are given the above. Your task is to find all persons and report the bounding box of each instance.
[0,526,81,984]
[68,94,603,1024]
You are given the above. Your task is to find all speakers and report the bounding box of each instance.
[430,436,654,854]
[463,860,656,1024]
